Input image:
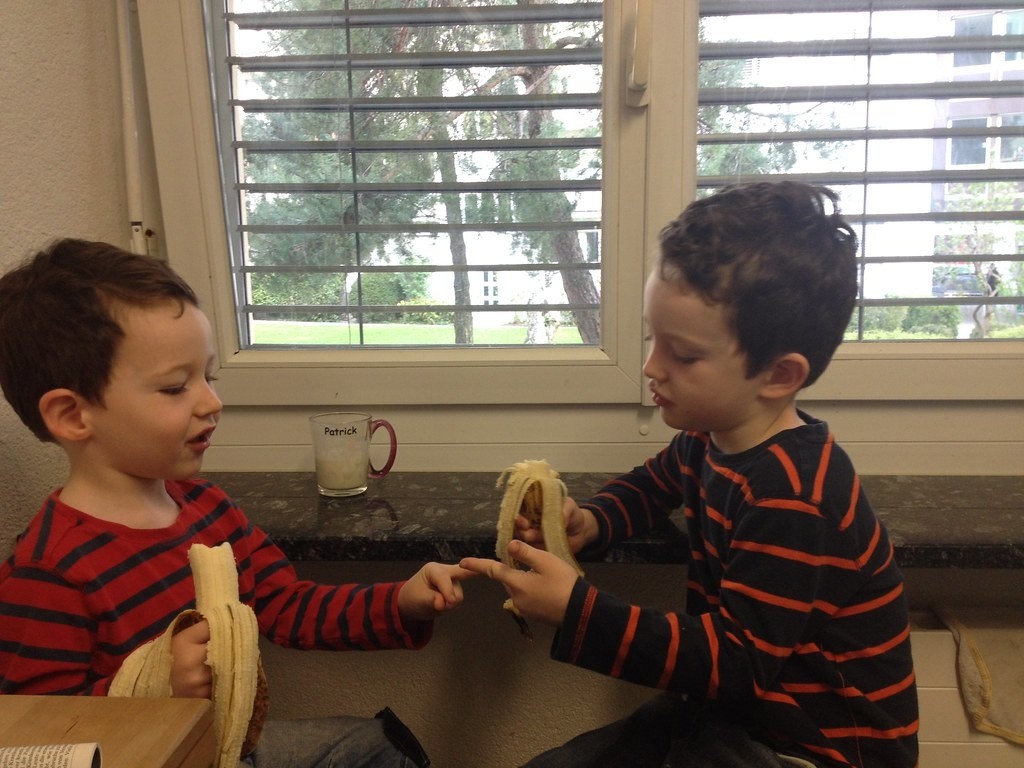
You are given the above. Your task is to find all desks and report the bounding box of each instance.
[0,694,218,768]
[188,470,1024,569]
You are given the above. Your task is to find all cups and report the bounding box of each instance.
[308,411,397,497]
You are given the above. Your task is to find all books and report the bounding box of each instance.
[0,742,101,768]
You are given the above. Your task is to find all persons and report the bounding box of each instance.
[1,234,484,768]
[454,179,921,768]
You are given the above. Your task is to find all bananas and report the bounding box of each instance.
[495,457,584,644]
[109,542,258,768]
